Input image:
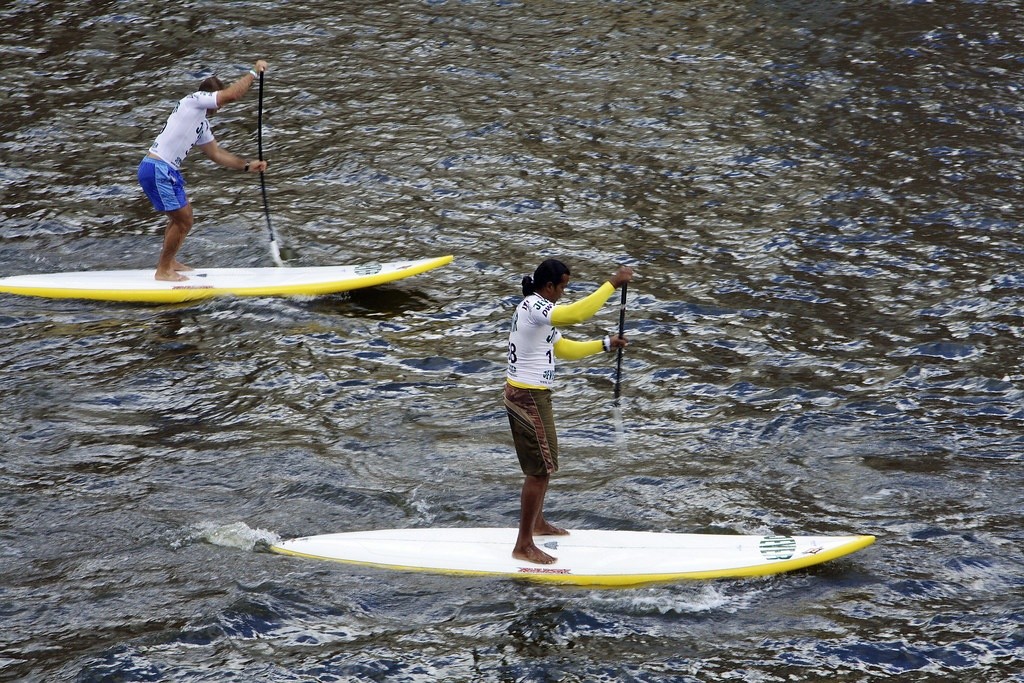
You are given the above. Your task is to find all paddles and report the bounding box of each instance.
[612,280,628,450]
[258,71,285,267]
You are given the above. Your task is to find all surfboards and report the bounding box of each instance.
[267,526,878,586]
[0,255,454,304]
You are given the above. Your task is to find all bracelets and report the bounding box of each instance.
[602,334,611,351]
[609,280,616,288]
[245,162,250,172]
[249,70,260,79]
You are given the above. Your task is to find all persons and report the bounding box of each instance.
[137,59,269,281]
[503,260,634,563]
